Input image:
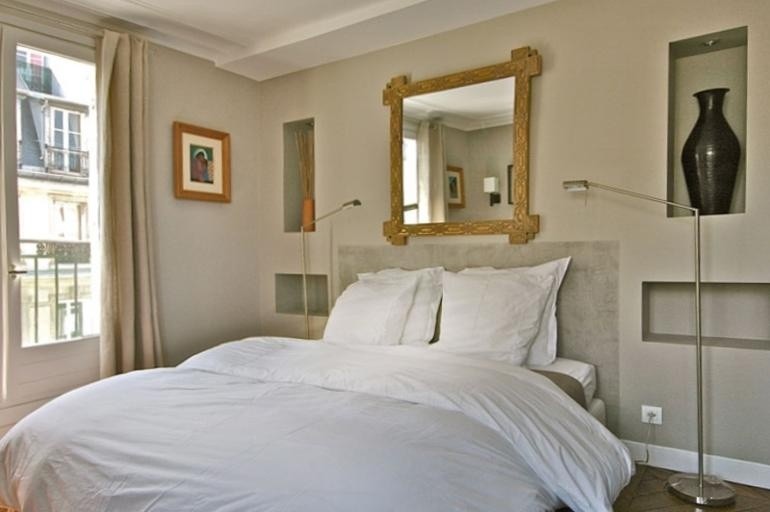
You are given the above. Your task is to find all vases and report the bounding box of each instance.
[681,86,743,214]
[298,199,316,233]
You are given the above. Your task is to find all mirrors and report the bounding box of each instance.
[382,45,543,247]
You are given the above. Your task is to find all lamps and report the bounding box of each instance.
[562,176,738,506]
[290,198,363,339]
[483,177,501,207]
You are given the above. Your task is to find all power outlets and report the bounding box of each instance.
[638,404,665,426]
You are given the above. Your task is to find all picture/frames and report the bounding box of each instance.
[507,164,514,205]
[445,163,469,212]
[171,118,231,206]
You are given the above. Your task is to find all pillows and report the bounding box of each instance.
[435,269,555,364]
[523,255,570,366]
[320,270,417,346]
[399,267,440,345]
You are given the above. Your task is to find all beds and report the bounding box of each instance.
[1,333,634,511]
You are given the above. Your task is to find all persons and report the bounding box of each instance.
[191,151,210,183]
